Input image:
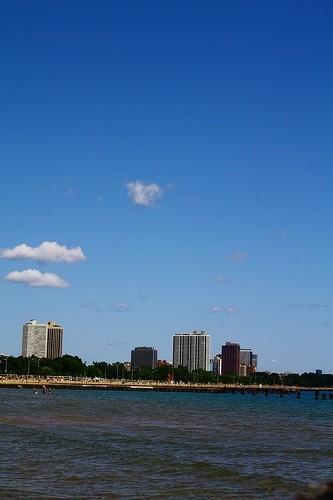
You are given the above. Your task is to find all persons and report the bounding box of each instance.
[33,387,37,395]
[49,388,51,394]
[43,385,46,397]
